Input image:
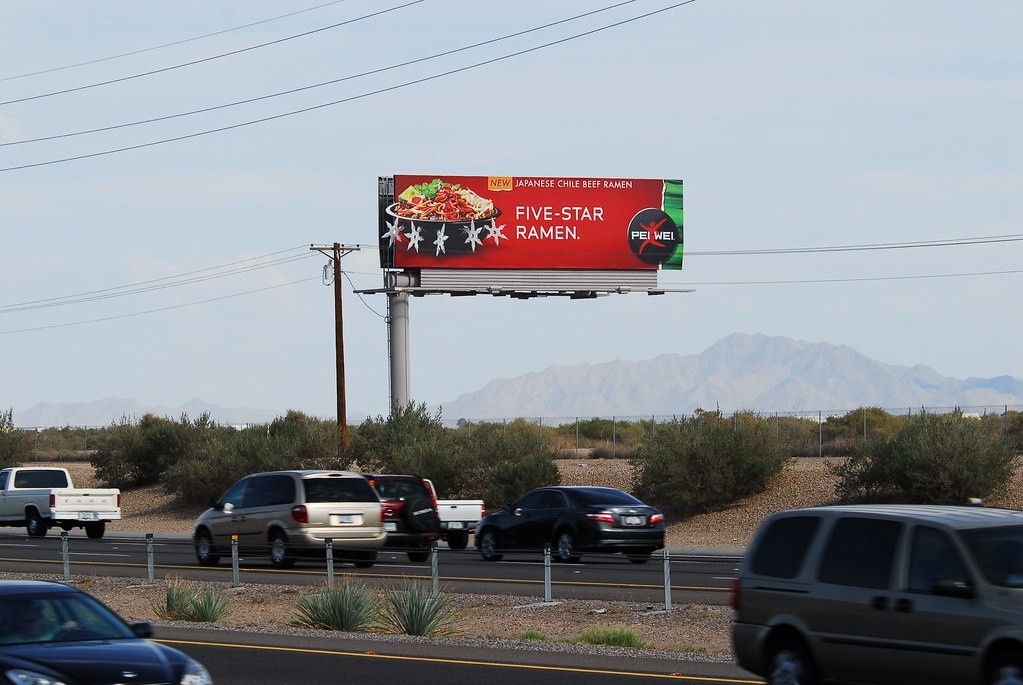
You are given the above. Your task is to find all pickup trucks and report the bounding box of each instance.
[0,467,123,539]
[421,478,486,550]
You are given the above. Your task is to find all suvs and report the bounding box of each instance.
[364,474,441,562]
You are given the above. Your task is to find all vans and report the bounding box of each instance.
[192,468,390,572]
[727,500,1022,685]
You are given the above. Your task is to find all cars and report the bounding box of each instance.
[0,577,213,685]
[473,485,667,563]
